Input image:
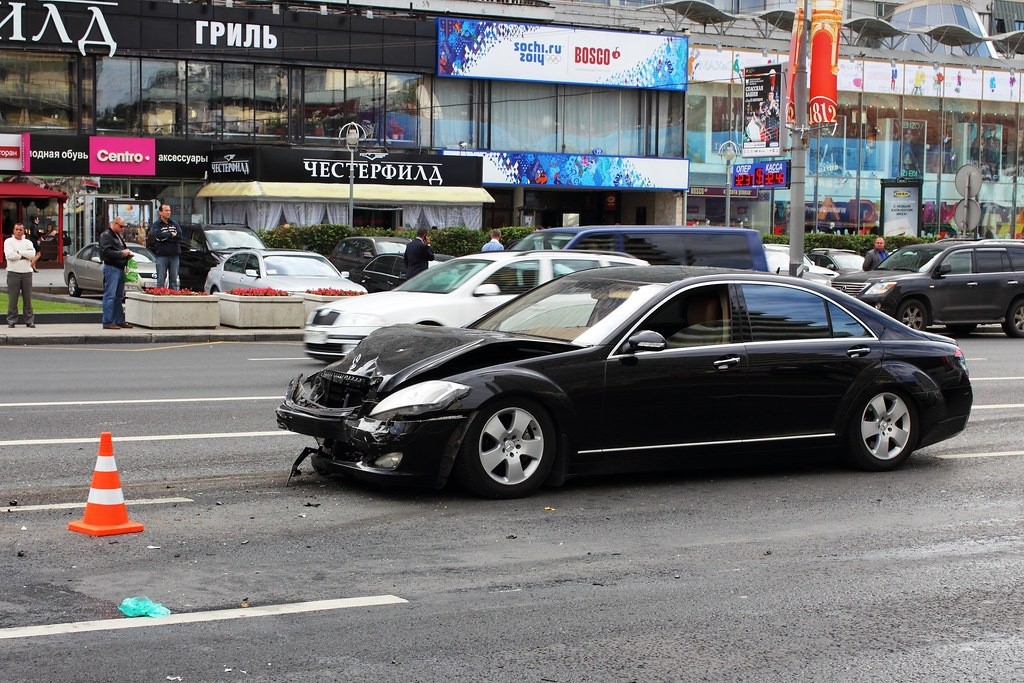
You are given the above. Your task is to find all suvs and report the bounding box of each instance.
[831,238,1024,338]
[805,249,867,274]
[762,242,840,279]
[302,251,651,363]
[178,222,268,292]
[330,235,412,270]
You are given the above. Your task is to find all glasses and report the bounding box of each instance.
[116,222,128,228]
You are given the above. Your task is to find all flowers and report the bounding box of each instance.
[308,287,363,299]
[142,286,208,296]
[226,287,283,297]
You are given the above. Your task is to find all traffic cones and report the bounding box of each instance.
[69,432,146,536]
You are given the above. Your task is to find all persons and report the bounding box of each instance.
[530,226,552,249]
[762,92,780,139]
[41,223,56,241]
[99,217,134,329]
[970,125,1000,181]
[148,202,183,290]
[862,237,894,270]
[404,227,434,281]
[482,229,504,251]
[110,220,148,246]
[30,215,44,273]
[3,222,36,328]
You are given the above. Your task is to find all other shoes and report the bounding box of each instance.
[103,324,121,329]
[8,324,15,328]
[33,268,39,272]
[26,323,36,328]
[116,323,133,328]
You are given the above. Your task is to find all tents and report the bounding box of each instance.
[0,182,68,263]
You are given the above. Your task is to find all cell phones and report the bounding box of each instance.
[423,234,426,240]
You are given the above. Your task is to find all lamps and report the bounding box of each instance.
[855,50,865,59]
[717,43,723,53]
[933,62,939,70]
[848,55,855,62]
[133,183,141,197]
[889,59,897,67]
[1009,68,1015,75]
[762,48,769,57]
[972,66,978,75]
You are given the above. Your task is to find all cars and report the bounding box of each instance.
[63,241,169,296]
[203,248,368,293]
[274,264,972,495]
[341,248,460,293]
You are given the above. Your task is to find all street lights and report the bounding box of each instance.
[718,142,741,226]
[339,122,367,230]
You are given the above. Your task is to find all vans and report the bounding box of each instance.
[513,224,767,272]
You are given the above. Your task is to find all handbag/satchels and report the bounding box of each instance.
[123,259,138,283]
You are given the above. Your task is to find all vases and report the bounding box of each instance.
[211,292,307,328]
[123,292,221,328]
[304,295,358,316]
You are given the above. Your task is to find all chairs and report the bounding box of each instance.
[518,269,536,294]
[686,296,719,326]
